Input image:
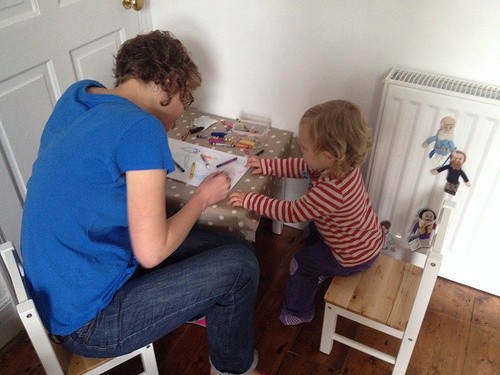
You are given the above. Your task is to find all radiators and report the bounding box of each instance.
[364,66,500,256]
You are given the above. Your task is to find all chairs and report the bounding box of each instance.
[0,241,159,375]
[321,200,455,375]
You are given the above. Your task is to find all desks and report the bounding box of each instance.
[159,108,294,241]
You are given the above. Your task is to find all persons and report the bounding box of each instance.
[20,30,270,375]
[228,100,384,325]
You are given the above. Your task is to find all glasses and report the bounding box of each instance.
[184,83,195,108]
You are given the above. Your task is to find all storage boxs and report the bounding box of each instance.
[232,120,269,149]
